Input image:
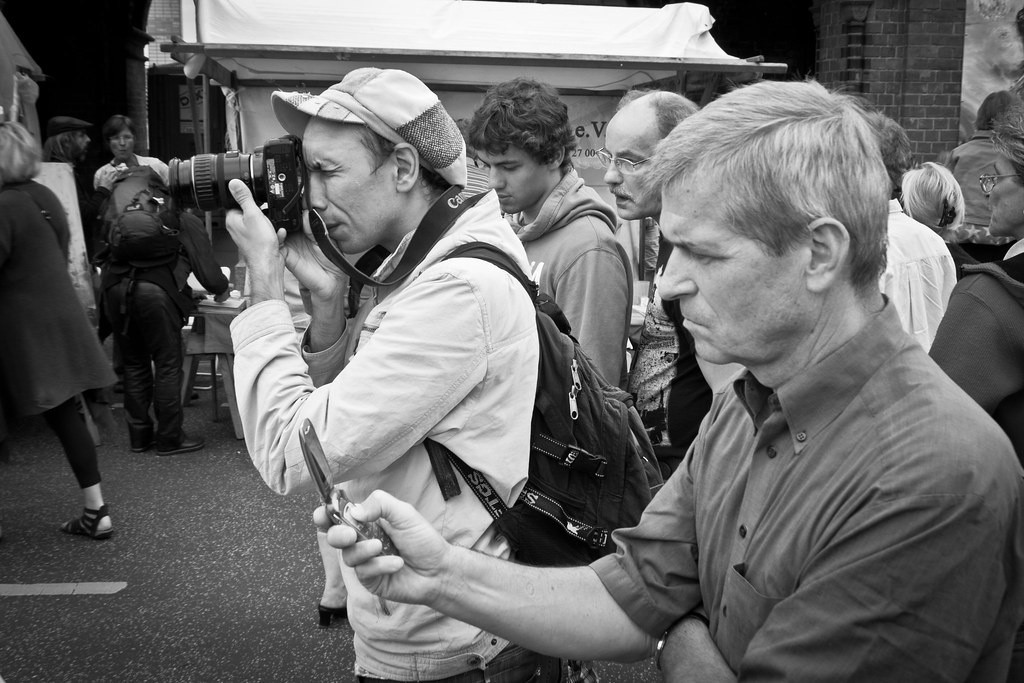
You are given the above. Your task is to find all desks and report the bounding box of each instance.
[178,295,246,438]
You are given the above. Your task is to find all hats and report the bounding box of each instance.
[47,116,93,137]
[271,67,468,187]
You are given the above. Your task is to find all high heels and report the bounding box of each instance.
[319,600,345,629]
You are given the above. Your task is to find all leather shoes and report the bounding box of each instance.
[155,435,205,456]
[131,442,156,452]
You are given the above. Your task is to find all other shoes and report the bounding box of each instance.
[191,391,200,400]
[114,379,127,394]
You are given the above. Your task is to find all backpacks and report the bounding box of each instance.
[103,165,179,261]
[423,242,664,568]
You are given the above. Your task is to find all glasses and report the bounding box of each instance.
[596,147,651,175]
[980,173,1020,194]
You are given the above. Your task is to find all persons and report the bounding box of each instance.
[313,79,1024,683]
[595,89,745,478]
[227,67,540,683]
[0,120,114,537]
[877,89,1024,469]
[43,114,230,456]
[468,76,633,392]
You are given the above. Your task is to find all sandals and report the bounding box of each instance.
[59,504,114,540]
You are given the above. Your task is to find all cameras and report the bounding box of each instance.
[167,136,304,233]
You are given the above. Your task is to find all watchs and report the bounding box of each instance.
[654,611,711,670]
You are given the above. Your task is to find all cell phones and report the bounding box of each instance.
[299,417,399,557]
